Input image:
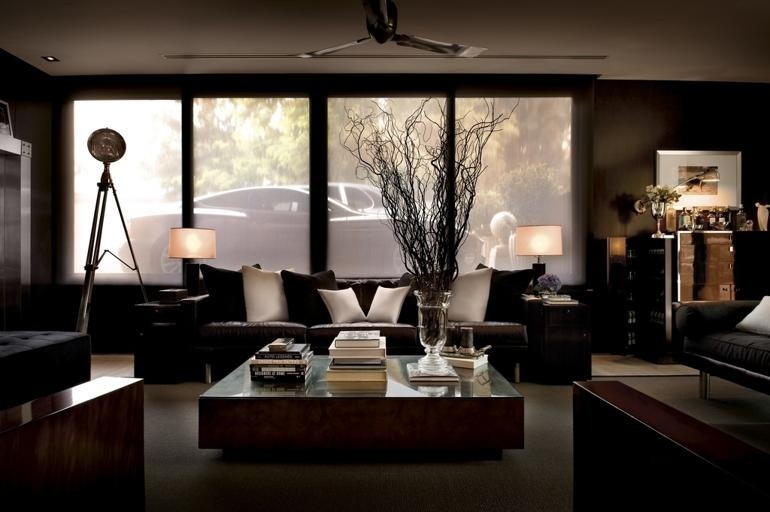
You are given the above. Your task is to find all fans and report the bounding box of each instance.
[289,0,488,59]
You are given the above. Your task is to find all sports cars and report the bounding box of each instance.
[118,184,488,281]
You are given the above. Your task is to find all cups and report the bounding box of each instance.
[461,327,474,348]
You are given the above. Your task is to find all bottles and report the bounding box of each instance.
[666,202,677,231]
[678,204,753,231]
[648,309,665,324]
[626,248,665,257]
[626,270,637,344]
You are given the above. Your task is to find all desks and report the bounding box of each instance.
[677,228,735,303]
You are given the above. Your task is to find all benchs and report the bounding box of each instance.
[0,330,91,410]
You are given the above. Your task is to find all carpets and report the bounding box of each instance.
[591,354,700,376]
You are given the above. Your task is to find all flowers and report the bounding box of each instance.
[644,183,681,203]
[537,273,562,292]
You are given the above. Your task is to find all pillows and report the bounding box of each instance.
[366,285,410,322]
[280,269,337,322]
[398,273,412,285]
[318,286,366,324]
[446,267,492,322]
[242,265,289,322]
[199,263,261,321]
[736,295,770,334]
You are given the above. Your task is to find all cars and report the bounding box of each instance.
[291,182,393,215]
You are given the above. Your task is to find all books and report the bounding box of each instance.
[249,330,387,386]
[326,387,387,398]
[405,358,459,382]
[250,387,310,396]
[443,354,489,369]
[542,294,578,305]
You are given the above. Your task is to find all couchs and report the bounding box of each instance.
[181,293,542,384]
[675,300,770,400]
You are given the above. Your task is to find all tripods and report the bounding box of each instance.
[75,168,150,333]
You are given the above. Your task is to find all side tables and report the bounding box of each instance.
[134,295,201,385]
[522,293,592,385]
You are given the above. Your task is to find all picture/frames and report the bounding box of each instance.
[656,149,743,213]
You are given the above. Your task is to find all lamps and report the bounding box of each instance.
[169,228,215,292]
[517,224,562,287]
[633,165,719,213]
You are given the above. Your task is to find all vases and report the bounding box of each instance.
[412,291,453,369]
[649,202,666,237]
